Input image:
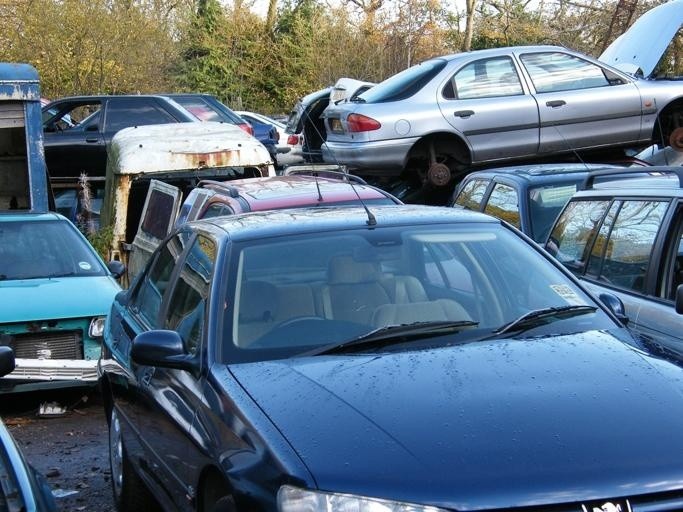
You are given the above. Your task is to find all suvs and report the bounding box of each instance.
[284,87,331,163]
[171,168,407,232]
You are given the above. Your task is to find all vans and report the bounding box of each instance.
[543,166,683,369]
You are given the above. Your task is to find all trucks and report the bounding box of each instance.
[76,122,277,291]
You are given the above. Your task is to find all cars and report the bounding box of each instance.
[0,211,125,403]
[41,94,202,189]
[452,164,683,248]
[240,113,279,146]
[158,94,253,139]
[235,110,305,167]
[318,0,683,194]
[101,206,650,512]
